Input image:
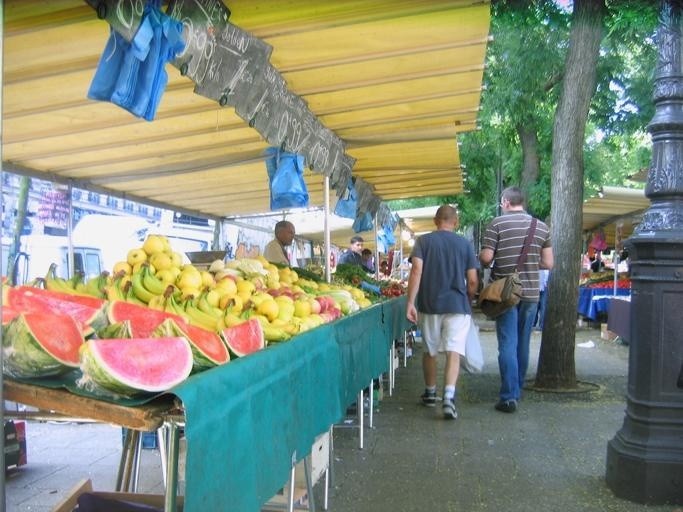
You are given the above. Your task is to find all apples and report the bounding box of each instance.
[113,234,371,331]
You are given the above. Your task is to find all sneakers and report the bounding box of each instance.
[495,398,517,413]
[442,399,458,418]
[420,394,437,408]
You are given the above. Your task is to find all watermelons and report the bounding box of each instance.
[1,285,263,397]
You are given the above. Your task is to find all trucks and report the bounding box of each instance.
[130,210,298,263]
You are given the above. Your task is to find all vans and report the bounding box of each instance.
[0,231,106,289]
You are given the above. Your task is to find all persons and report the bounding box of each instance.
[360,248,374,275]
[404,202,479,420]
[261,220,296,266]
[336,236,365,268]
[377,261,390,276]
[476,185,555,414]
[532,269,549,331]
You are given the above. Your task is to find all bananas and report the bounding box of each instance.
[1,263,290,347]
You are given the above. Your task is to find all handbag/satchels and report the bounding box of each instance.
[479,275,522,318]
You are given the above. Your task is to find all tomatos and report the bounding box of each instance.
[588,278,630,289]
[382,285,403,296]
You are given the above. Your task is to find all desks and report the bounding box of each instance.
[605,296,632,345]
[1,286,406,512]
[577,287,632,329]
